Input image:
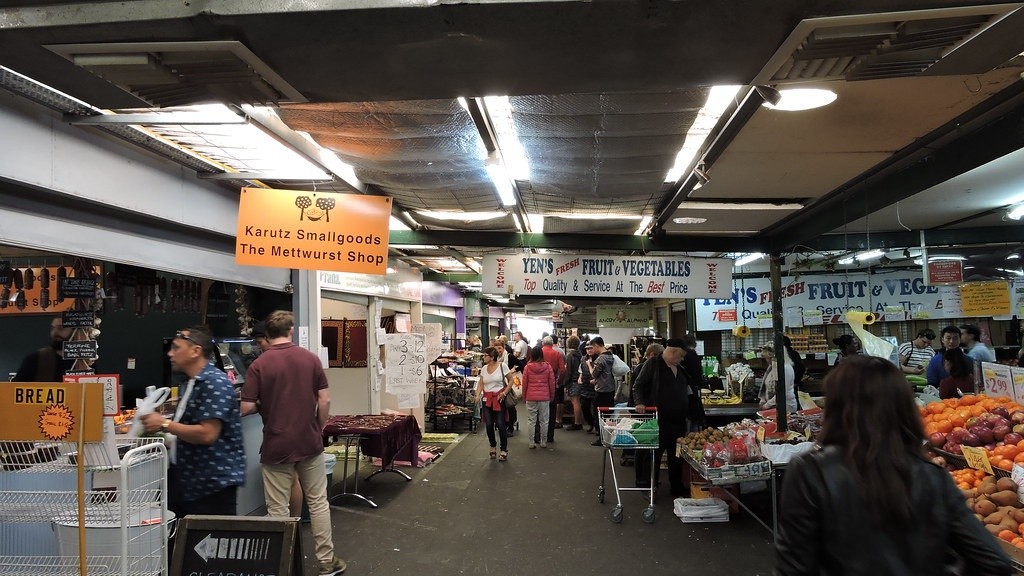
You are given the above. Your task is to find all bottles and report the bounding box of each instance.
[701,355,719,378]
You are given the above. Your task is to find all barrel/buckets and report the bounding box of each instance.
[51,503,176,570]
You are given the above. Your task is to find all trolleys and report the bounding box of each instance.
[596,406,660,523]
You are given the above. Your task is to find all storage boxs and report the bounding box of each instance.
[673,432,817,523]
[933,446,1024,567]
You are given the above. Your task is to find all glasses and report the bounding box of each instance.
[761,345,771,351]
[176,330,202,347]
[920,338,931,345]
[484,354,490,357]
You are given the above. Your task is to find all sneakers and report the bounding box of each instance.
[318,556,347,576]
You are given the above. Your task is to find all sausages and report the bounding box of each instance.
[0,261,200,318]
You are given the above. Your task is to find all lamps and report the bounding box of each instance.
[793,201,1024,276]
[485,157,516,206]
[694,168,712,185]
[756,85,781,104]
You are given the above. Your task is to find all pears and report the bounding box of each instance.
[962,474,1024,535]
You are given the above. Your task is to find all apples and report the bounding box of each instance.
[929,405,1024,467]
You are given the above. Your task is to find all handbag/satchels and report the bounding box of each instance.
[500,363,518,407]
[611,352,631,377]
[129,385,171,438]
[505,370,523,399]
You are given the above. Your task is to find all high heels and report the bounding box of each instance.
[498,452,508,461]
[489,446,496,459]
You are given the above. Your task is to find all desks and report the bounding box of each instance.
[428,375,483,433]
[322,414,422,507]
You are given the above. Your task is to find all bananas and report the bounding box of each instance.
[701,395,742,404]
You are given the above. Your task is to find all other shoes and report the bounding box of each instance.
[587,425,594,431]
[539,442,547,448]
[565,423,583,431]
[620,458,635,466]
[554,421,562,429]
[507,431,513,437]
[642,490,656,501]
[547,439,554,442]
[590,437,603,446]
[595,432,600,436]
[529,443,536,449]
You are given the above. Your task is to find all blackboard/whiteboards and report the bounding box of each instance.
[63,340,97,360]
[170,515,306,576]
[65,368,95,376]
[59,277,96,298]
[62,310,94,328]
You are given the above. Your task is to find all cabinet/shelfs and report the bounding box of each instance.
[0,437,168,576]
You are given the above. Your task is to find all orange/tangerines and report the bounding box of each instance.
[998,523,1024,551]
[916,394,1024,435]
[949,467,989,494]
[983,440,1024,472]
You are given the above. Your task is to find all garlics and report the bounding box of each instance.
[234,289,253,336]
[88,274,104,361]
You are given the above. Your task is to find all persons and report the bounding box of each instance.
[470,331,532,437]
[475,348,514,460]
[141,324,248,517]
[758,336,805,415]
[632,337,690,501]
[522,346,555,449]
[940,349,974,398]
[537,332,623,446]
[926,326,975,388]
[683,335,706,427]
[534,336,566,444]
[774,354,1013,576]
[241,310,346,576]
[832,335,856,366]
[12,314,75,382]
[899,329,936,375]
[621,342,665,458]
[958,325,993,361]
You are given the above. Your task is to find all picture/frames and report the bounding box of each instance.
[321,319,344,367]
[344,319,367,367]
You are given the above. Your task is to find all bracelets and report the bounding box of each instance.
[160,420,171,432]
[503,392,506,396]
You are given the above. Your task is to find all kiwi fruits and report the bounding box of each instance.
[676,427,730,450]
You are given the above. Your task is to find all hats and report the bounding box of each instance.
[583,340,594,348]
[540,335,553,345]
[665,338,692,353]
